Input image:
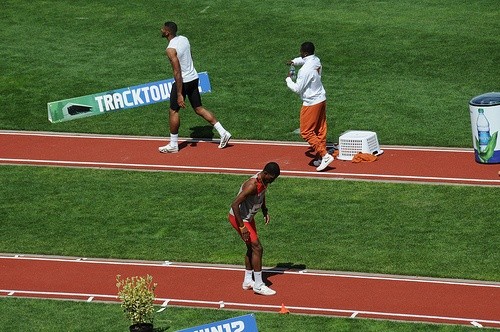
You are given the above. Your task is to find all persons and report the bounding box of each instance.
[228,161,281,295]
[158,22,231,153]
[285,42,334,171]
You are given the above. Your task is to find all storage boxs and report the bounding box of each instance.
[336,130,383,161]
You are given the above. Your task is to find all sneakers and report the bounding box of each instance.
[313,160,321,166]
[159,145,178,154]
[317,154,334,171]
[218,131,232,149]
[253,283,276,295]
[243,281,255,289]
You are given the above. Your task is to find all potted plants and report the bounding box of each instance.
[116,273,158,332]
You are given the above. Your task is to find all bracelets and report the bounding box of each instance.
[239,225,245,230]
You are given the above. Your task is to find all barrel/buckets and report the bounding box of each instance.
[469,91,500,163]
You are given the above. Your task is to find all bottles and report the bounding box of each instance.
[289,64,296,81]
[477,108,490,153]
